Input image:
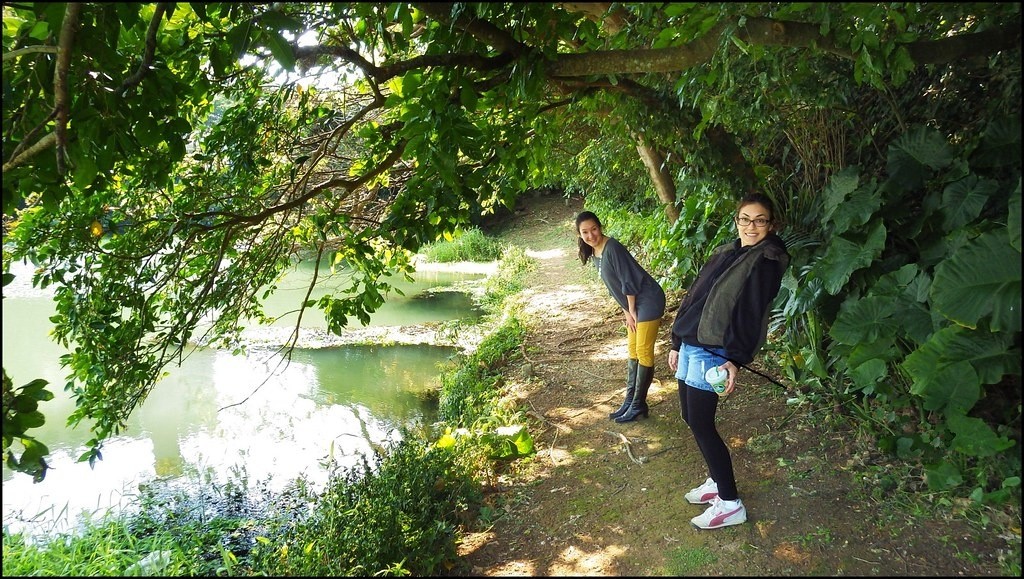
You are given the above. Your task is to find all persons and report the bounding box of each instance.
[667,193,792,531]
[575,211,665,425]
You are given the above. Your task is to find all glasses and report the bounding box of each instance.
[736,217,771,227]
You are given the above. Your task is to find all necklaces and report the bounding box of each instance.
[598,243,605,284]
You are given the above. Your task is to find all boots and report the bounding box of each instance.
[609,358,640,419]
[613,362,655,422]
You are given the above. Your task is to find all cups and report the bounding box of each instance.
[705,366,729,396]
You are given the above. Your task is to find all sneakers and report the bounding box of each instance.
[690,495,747,530]
[684,477,719,504]
